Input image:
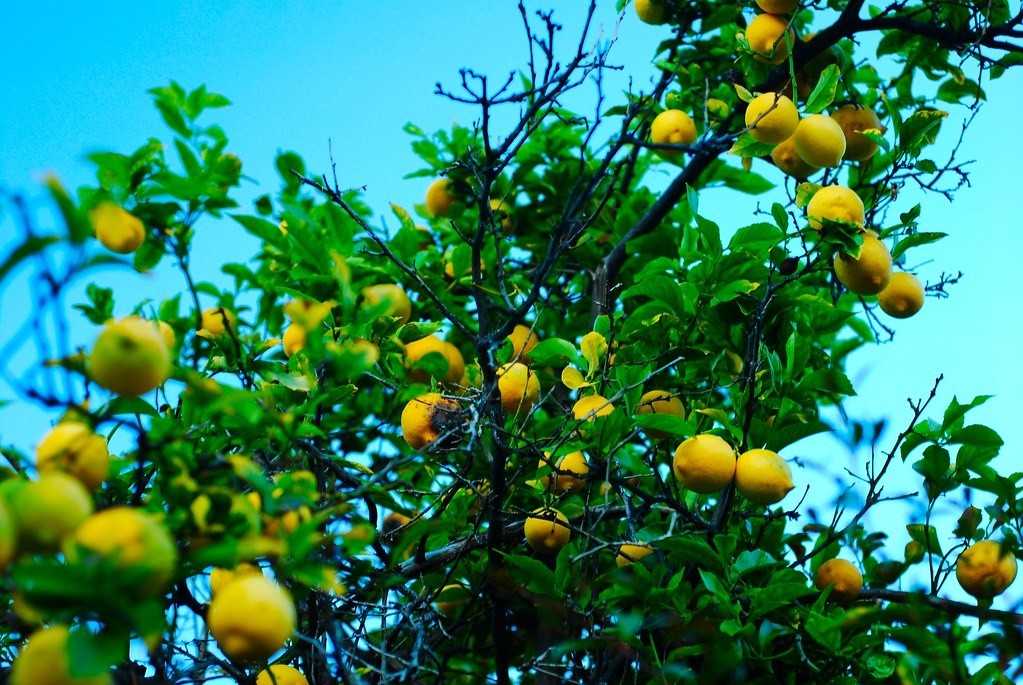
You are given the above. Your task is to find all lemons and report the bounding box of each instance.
[0,1,927,685]
[956,540,1016,599]
